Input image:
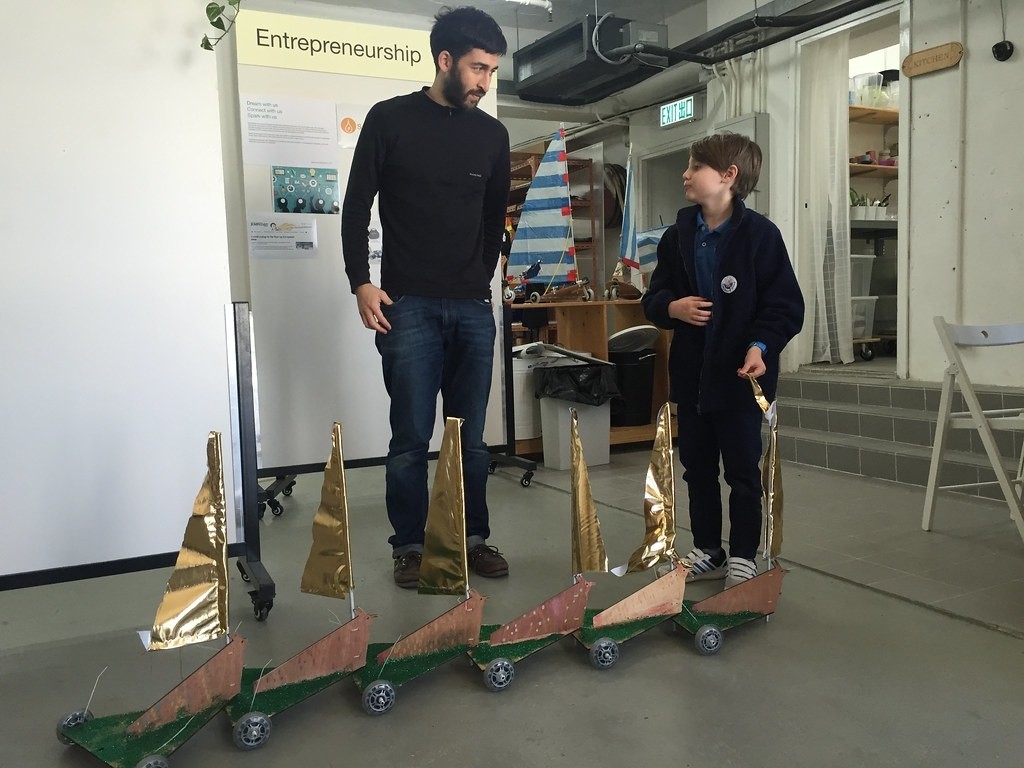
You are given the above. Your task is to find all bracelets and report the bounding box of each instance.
[746,341,769,360]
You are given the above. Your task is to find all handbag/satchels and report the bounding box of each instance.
[607,280,642,300]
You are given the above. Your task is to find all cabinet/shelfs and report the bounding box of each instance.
[505,300,677,445]
[506,156,600,301]
[850,103,899,234]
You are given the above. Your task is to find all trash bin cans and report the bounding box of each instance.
[533,341,615,471]
[512,341,542,441]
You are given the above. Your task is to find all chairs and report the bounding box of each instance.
[921,317,1024,545]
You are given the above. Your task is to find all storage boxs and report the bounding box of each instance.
[511,140,549,166]
[849,254,899,339]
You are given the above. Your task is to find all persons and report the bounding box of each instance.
[641,133,805,590]
[340,5,513,591]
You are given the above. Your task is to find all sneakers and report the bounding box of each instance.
[393,550,422,587]
[468,543,509,578]
[724,557,757,591]
[656,548,729,583]
